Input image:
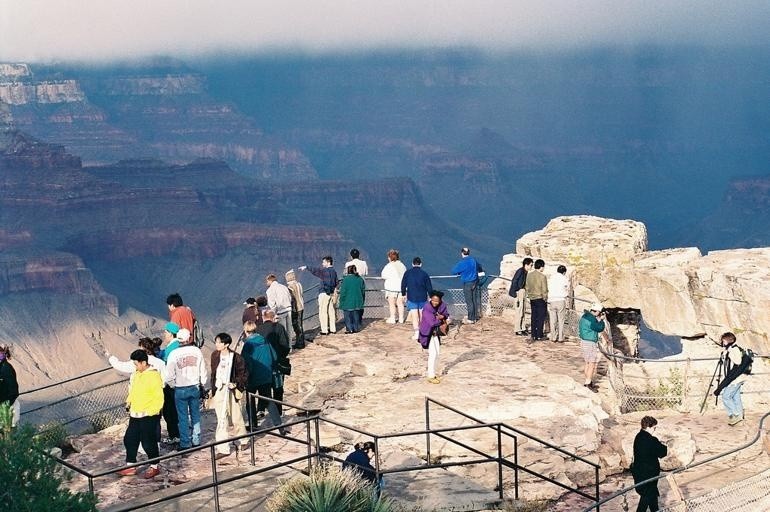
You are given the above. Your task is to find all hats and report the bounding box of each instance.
[161,322,190,342]
[591,303,603,312]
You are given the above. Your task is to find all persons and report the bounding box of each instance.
[297,249,368,335]
[508,258,569,343]
[632,416,667,512]
[579,306,605,393]
[381,249,452,384]
[722,332,747,425]
[210,269,304,460]
[448,249,485,324]
[0,344,20,427]
[105,292,208,479]
[342,441,383,498]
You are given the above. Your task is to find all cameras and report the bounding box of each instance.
[600,307,609,316]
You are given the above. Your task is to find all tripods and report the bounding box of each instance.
[700,352,724,414]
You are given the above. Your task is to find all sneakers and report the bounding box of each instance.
[516,331,528,336]
[140,467,159,478]
[427,378,439,384]
[116,464,136,475]
[584,381,599,393]
[728,416,743,426]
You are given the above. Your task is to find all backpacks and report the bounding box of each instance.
[193,320,204,348]
